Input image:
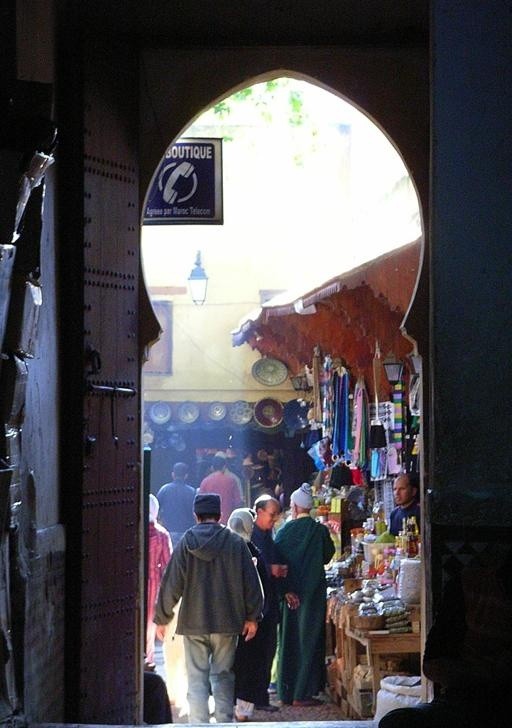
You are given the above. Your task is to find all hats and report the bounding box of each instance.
[192,493,221,515]
[290,482,313,510]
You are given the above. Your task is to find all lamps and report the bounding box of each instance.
[187,251,209,306]
[383,349,404,385]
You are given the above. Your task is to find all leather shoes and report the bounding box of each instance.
[292,697,325,707]
[256,704,279,712]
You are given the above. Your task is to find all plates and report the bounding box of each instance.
[177,400,199,424]
[207,401,227,421]
[282,400,312,430]
[229,399,253,425]
[254,397,284,428]
[150,401,174,426]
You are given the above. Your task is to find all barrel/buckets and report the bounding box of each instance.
[362,541,395,563]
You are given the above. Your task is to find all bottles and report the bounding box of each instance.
[311,484,348,514]
[351,514,420,586]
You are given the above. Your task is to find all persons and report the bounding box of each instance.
[154,448,248,549]
[274,482,336,708]
[234,494,302,713]
[147,493,174,663]
[153,491,265,722]
[388,472,420,538]
[142,649,174,724]
[206,506,281,722]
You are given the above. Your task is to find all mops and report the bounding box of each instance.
[368,353,388,449]
